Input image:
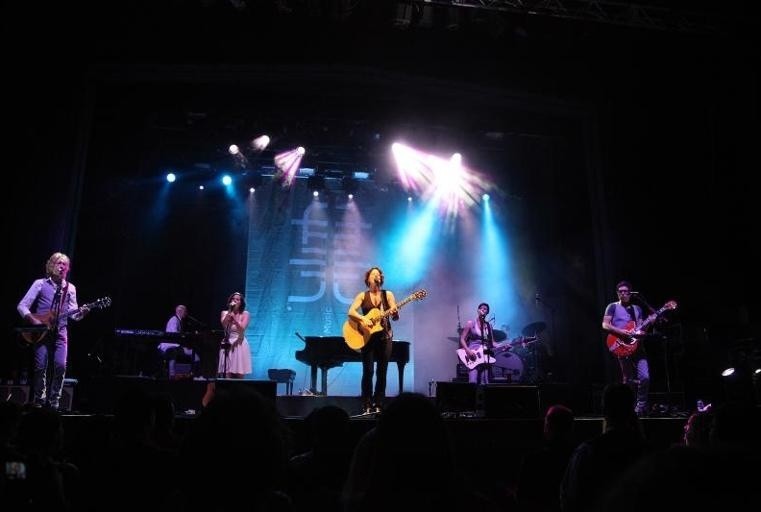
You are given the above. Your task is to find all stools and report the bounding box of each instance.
[269,369,295,397]
[168,362,190,380]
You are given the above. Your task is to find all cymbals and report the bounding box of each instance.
[456,328,463,333]
[448,336,460,342]
[521,321,546,336]
[493,330,507,342]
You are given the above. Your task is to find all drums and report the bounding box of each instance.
[514,344,534,356]
[489,350,525,383]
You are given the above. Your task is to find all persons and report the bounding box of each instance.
[458,302,513,384]
[216,291,253,379]
[156,302,202,378]
[1,368,761,511]
[15,251,93,410]
[601,280,658,418]
[348,267,402,416]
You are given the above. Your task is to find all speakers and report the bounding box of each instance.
[215,378,276,408]
[485,384,540,417]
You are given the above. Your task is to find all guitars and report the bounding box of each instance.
[343,289,426,350]
[607,300,678,359]
[457,336,537,370]
[21,296,112,345]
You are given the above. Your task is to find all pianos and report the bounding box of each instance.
[295,336,409,398]
[115,328,204,347]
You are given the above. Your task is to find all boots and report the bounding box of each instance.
[362,391,385,413]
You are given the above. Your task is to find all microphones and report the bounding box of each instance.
[229,300,236,312]
[374,275,379,284]
[627,291,640,295]
[477,309,486,315]
[56,266,66,276]
